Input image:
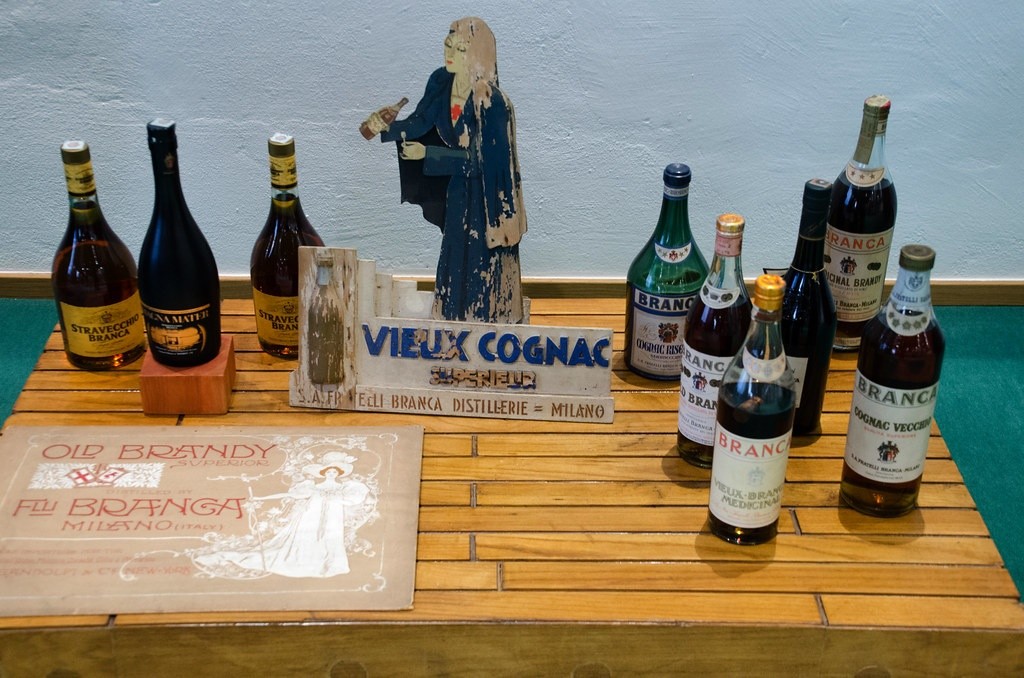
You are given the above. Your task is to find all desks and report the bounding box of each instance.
[0,273,1024,678]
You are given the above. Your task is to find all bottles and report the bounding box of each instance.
[250,131,325,358]
[624,162,838,532]
[359,97,410,141]
[52,115,225,370]
[840,244,947,519]
[823,90,899,348]
